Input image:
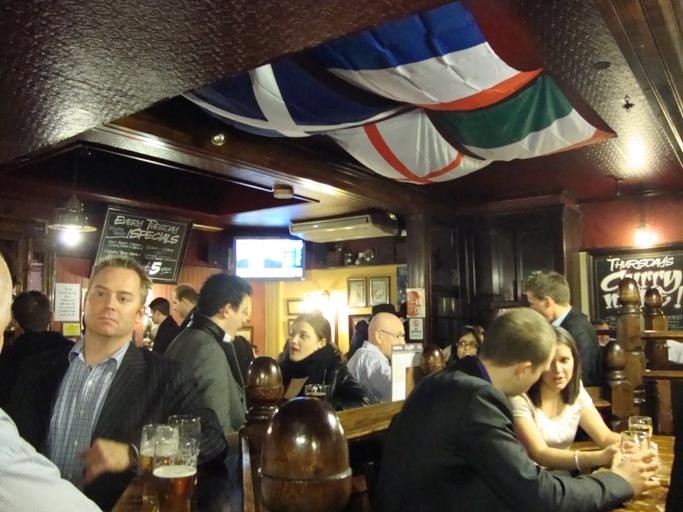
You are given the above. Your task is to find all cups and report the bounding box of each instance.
[303,383,326,403]
[620,430,654,497]
[134,413,203,512]
[627,416,654,450]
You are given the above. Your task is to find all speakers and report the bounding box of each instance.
[205,242,221,265]
[315,246,327,264]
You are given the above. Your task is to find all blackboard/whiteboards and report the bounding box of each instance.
[90,204,194,285]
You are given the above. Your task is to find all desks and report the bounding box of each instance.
[528,427,678,512]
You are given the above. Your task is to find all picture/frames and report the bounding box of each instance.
[367,275,390,307]
[345,276,367,308]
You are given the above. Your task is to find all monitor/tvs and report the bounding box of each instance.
[232,235,306,282]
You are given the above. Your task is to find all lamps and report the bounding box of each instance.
[44,190,101,253]
